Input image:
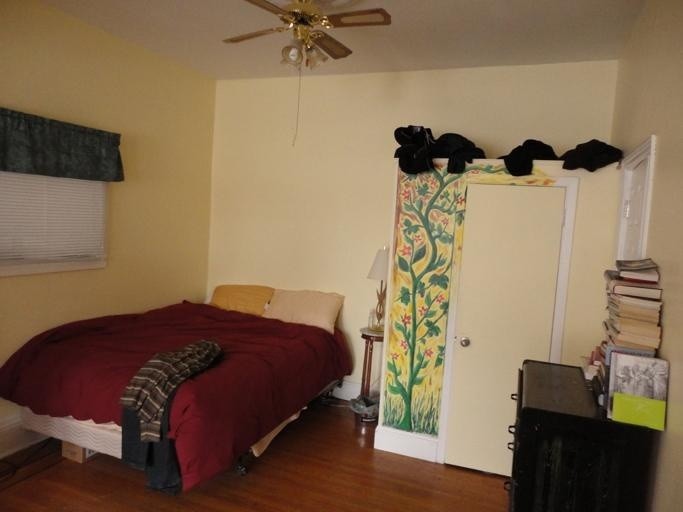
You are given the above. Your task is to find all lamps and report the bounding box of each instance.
[366,249,389,333]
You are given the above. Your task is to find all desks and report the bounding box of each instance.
[352,326,384,428]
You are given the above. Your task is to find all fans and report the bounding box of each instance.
[222,1,392,147]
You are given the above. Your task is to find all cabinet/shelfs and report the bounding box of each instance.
[508,359,655,512]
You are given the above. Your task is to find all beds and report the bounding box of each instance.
[5,301,353,490]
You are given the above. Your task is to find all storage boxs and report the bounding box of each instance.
[61,441,100,464]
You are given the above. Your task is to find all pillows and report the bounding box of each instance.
[209,282,273,316]
[266,289,343,334]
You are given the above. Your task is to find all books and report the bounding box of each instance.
[577,257,670,433]
